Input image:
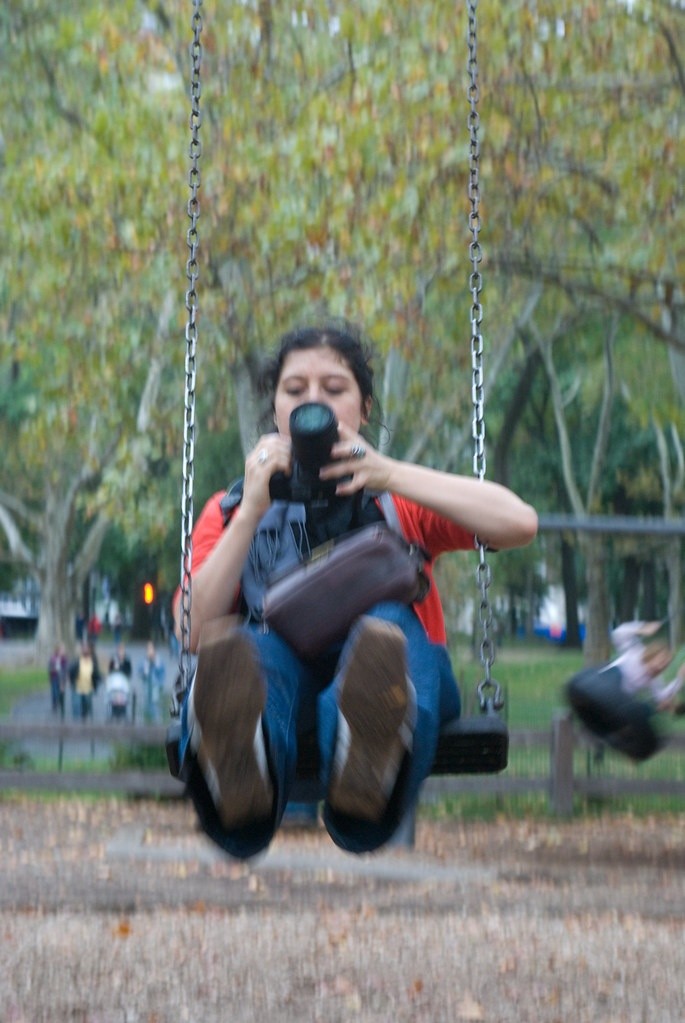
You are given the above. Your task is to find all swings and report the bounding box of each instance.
[163,1,511,784]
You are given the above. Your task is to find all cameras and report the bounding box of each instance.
[269,402,353,504]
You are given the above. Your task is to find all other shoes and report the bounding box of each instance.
[188,627,279,830]
[330,615,416,817]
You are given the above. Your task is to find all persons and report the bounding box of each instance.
[581,620,685,758]
[107,645,131,717]
[50,643,67,713]
[76,611,101,643]
[114,613,122,644]
[172,329,537,855]
[139,640,166,717]
[67,641,100,717]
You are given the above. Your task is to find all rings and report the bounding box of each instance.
[352,444,365,458]
[257,451,266,464]
